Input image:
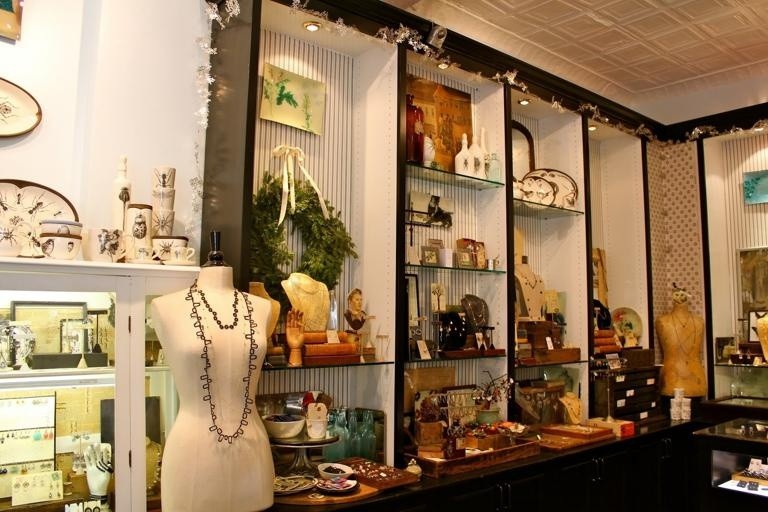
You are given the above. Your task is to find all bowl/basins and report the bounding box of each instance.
[317,462,353,480]
[39,220,82,260]
[261,414,305,439]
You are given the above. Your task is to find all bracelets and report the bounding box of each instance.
[95,461,105,473]
[99,458,114,474]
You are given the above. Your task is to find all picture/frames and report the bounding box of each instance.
[405,274,421,340]
[748,310,768,343]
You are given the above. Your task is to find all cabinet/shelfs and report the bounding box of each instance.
[550,438,635,512]
[586,114,654,419]
[510,86,592,423]
[693,415,768,512]
[400,45,512,465]
[435,458,550,512]
[0,255,201,512]
[635,424,692,512]
[697,123,768,401]
[199,0,399,473]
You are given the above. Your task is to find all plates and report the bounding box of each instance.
[522,176,556,209]
[0,78,43,137]
[610,308,642,342]
[315,479,357,492]
[0,178,80,258]
[273,475,317,496]
[162,260,196,265]
[129,259,161,265]
[522,168,579,209]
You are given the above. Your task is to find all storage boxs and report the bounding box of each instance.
[589,364,664,421]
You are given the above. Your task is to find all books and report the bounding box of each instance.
[594,329,621,354]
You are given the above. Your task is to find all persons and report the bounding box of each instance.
[624,321,637,347]
[286,309,305,368]
[84,444,112,496]
[655,288,708,398]
[157,231,274,512]
[343,289,367,329]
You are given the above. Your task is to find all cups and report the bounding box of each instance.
[124,166,195,261]
[305,420,329,438]
[670,387,693,420]
[87,228,135,263]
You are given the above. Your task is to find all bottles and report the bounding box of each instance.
[323,408,376,461]
[454,127,501,187]
[406,92,425,167]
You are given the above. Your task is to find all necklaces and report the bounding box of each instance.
[147,441,161,491]
[564,397,581,419]
[671,311,697,356]
[518,267,538,289]
[592,303,607,319]
[465,297,486,326]
[288,277,326,332]
[448,318,465,338]
[197,288,238,332]
[187,279,258,442]
